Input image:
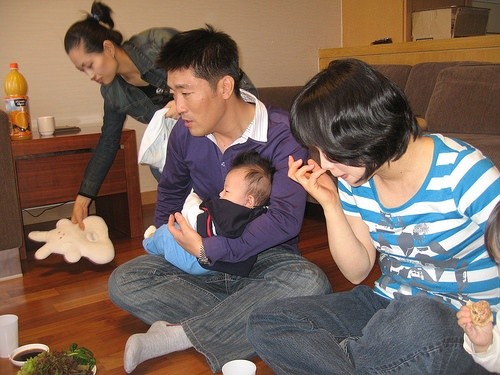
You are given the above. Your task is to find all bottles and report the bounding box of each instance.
[3,62,33,140]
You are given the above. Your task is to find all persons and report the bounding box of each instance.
[247,59,500,375]
[107,23,334,375]
[64,0,259,231]
[456,200,500,374]
[143,152,273,275]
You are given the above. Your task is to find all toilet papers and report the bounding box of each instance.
[0,313,19,359]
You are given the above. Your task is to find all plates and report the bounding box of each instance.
[63,349,97,375]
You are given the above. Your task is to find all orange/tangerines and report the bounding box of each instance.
[15,112,29,129]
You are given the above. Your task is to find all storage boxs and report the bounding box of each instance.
[410,4,490,41]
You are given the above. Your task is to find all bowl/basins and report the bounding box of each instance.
[8,343,50,366]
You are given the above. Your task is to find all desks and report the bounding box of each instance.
[11,125,142,260]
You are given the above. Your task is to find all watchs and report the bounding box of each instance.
[199,246,210,264]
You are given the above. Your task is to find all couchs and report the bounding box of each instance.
[258,60,500,205]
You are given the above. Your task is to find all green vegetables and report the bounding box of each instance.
[17,343,96,375]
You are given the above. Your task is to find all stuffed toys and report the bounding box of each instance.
[28,217,115,265]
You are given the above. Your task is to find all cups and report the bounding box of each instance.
[221,359,257,375]
[0,314,19,359]
[37,116,55,136]
